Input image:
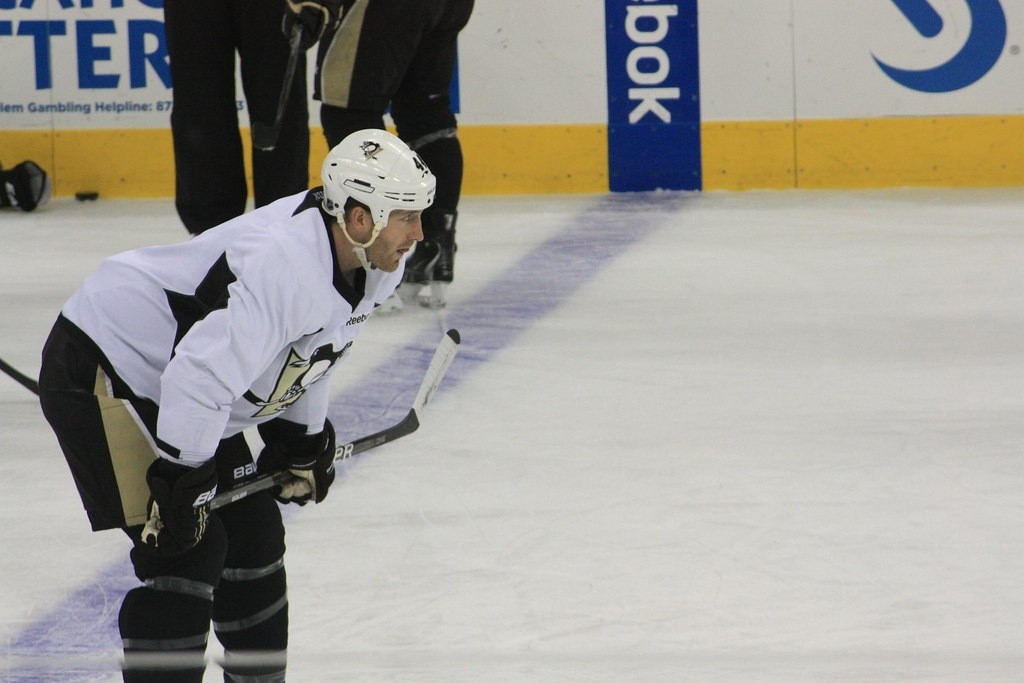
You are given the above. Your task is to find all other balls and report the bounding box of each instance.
[73,190,97,201]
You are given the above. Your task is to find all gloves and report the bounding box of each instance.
[263,421,338,506]
[142,459,214,555]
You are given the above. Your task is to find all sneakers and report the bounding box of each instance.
[401,206,457,307]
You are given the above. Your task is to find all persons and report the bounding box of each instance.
[39,129,436,683]
[163,0,310,235]
[282,0,474,281]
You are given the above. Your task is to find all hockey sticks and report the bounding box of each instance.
[208,328,463,512]
[249,26,302,151]
[0,359,40,396]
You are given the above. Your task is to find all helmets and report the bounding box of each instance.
[320,130,438,252]
